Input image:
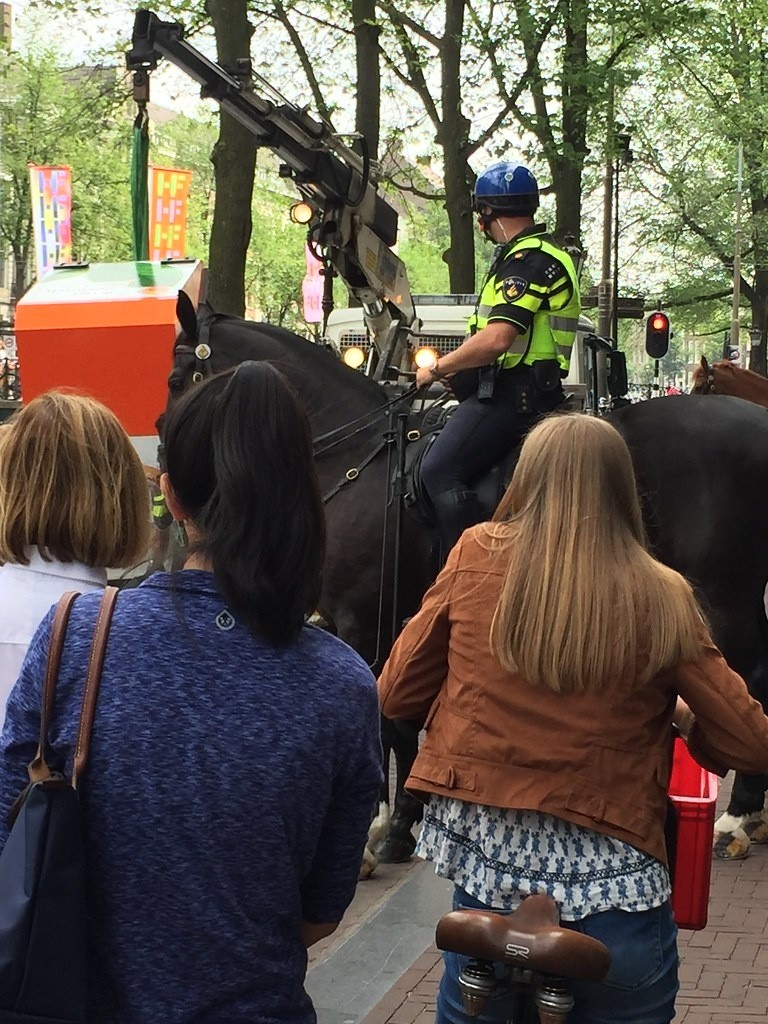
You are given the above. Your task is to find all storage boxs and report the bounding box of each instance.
[663,736,718,932]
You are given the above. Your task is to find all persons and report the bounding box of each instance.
[379,418,768,1024]
[0,361,383,1024]
[401,164,582,626]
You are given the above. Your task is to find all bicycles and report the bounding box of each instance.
[432,723,698,1024]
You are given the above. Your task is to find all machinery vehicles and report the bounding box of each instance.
[120,6,597,420]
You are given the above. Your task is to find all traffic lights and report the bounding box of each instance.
[645,311,670,362]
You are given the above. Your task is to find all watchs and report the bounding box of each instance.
[429,361,443,379]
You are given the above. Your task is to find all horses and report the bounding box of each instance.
[153,289,768,881]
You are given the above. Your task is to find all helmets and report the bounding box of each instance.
[475,165,541,214]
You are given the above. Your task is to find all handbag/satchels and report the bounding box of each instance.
[2,588,122,1023]
